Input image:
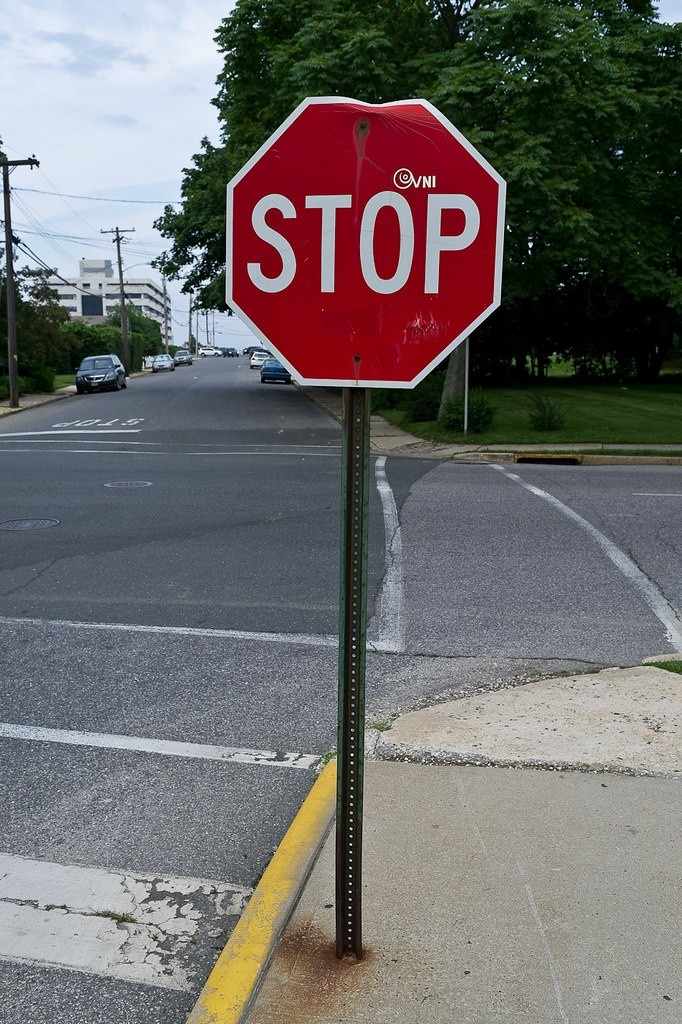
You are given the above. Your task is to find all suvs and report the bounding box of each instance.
[198,346,223,357]
[224,348,238,357]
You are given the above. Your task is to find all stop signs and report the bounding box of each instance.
[224,95,507,390]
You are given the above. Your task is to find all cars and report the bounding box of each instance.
[250,352,270,369]
[261,358,292,384]
[174,350,193,365]
[76,354,127,393]
[151,354,174,372]
[243,345,264,358]
